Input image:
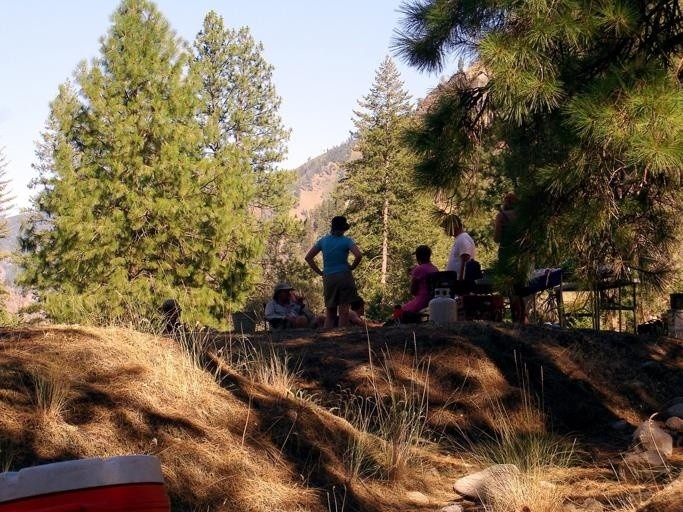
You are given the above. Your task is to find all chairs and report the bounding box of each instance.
[423,268,458,323]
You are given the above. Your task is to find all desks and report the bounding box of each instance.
[482,268,641,335]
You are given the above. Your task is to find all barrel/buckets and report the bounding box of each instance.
[233,312,256,331]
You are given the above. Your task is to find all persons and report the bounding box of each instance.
[344,295,382,328]
[438,214,476,300]
[261,283,325,330]
[161,298,186,335]
[492,192,530,325]
[390,245,440,320]
[304,215,361,331]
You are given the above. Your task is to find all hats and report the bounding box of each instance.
[274,282,295,293]
[411,244,432,256]
[330,214,351,230]
